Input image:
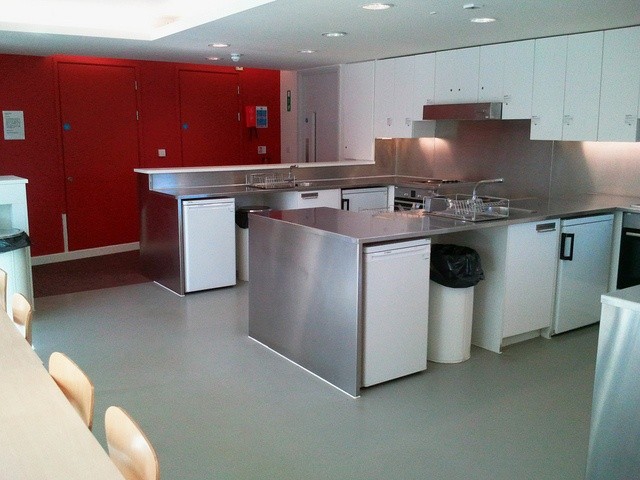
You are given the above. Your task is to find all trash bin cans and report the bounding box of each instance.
[0,229,33,313]
[234,206,273,282]
[429,243,485,363]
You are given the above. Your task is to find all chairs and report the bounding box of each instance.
[11,292,33,346]
[104,406,160,480]
[48,351,94,431]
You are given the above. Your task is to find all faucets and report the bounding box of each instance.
[473,178,503,200]
[288,164,298,178]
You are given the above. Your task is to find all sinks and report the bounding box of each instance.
[483,207,539,217]
[294,182,320,187]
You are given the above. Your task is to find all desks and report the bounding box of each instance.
[0,310,128,480]
